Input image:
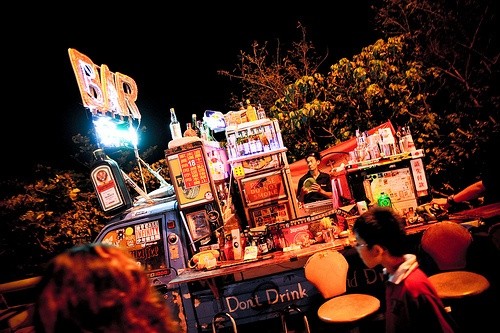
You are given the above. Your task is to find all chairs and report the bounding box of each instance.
[421,220,490,301]
[304,249,381,333]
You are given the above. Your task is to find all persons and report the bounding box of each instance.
[353,207,453,333]
[33,244,178,333]
[429,171,500,217]
[296,152,333,204]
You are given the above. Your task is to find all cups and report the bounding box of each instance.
[323,229,333,243]
[354,148,366,163]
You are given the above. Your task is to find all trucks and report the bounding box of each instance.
[88,198,500,332]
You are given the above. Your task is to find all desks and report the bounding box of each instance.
[168,203,470,333]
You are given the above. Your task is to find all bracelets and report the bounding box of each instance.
[447,194,461,206]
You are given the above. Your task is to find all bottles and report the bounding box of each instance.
[257,103,266,119]
[378,192,392,207]
[184,123,196,136]
[236,102,248,122]
[169,108,182,139]
[244,224,276,255]
[191,114,201,137]
[228,127,276,157]
[246,99,256,121]
[90,137,133,217]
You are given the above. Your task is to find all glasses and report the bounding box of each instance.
[354,241,371,250]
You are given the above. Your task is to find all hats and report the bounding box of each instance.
[305,152,320,160]
